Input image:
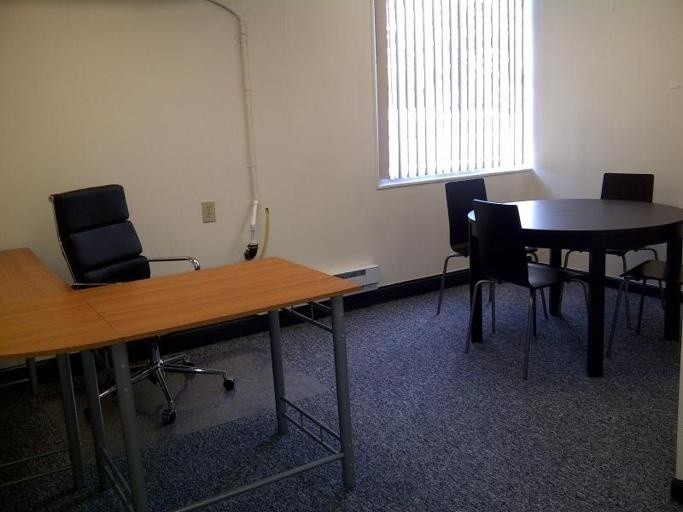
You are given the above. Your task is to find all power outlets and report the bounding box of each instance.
[201,201,215,223]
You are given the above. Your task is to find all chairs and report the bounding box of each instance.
[437,177,549,333]
[606,260,682,356]
[554,172,657,329]
[48,185,236,424]
[458,200,585,382]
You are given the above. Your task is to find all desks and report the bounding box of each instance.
[78,248,360,512]
[0,246,123,512]
[467,198,682,379]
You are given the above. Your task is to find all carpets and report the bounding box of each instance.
[42,351,330,464]
